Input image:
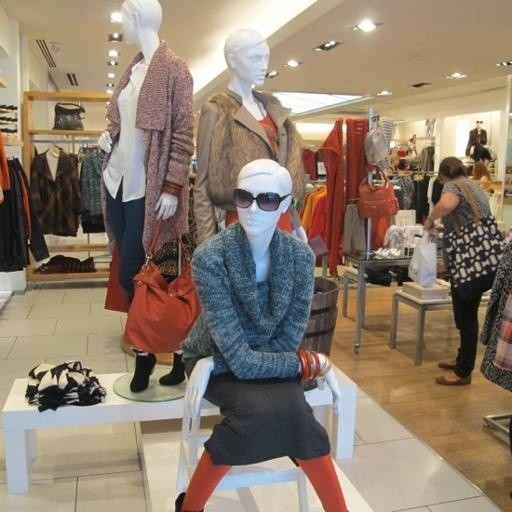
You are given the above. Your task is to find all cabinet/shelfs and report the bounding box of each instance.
[23,92,129,283]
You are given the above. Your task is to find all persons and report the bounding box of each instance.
[406,135,420,157]
[97,0,196,393]
[465,119,491,162]
[197,26,307,245]
[425,118,435,135]
[176,160,358,511]
[423,157,503,384]
[472,163,495,191]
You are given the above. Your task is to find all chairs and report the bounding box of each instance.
[175,354,308,512]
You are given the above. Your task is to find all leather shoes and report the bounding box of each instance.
[435,374,471,386]
[437,359,458,370]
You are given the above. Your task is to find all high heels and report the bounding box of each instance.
[158,354,186,388]
[128,354,154,393]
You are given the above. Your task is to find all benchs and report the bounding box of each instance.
[2,353,356,496]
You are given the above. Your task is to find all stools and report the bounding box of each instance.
[389,290,490,365]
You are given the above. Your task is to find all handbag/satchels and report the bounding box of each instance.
[443,180,508,289]
[408,229,437,287]
[120,213,200,353]
[52,102,86,130]
[358,166,399,218]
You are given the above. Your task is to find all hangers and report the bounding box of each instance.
[297,181,332,201]
[52,138,61,148]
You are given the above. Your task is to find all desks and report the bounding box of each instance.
[321,248,447,354]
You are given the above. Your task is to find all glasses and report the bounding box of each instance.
[232,188,293,212]
[476,121,483,123]
